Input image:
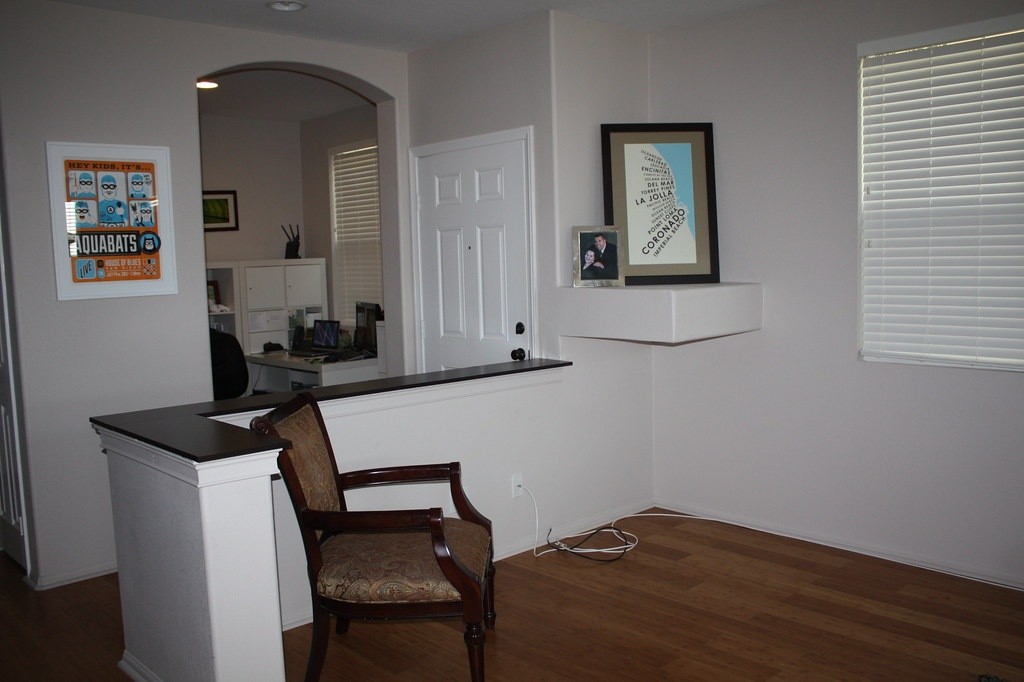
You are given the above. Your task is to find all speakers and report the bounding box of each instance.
[292,326,304,351]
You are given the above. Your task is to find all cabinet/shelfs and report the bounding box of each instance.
[207,256,329,356]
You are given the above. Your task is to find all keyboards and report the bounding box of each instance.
[288,350,324,357]
[335,351,356,360]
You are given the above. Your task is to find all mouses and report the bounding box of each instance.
[324,357,336,362]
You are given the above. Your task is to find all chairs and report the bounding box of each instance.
[250,390,497,682]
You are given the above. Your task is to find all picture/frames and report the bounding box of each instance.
[45,140,179,301]
[571,225,625,287]
[600,122,720,286]
[202,190,240,233]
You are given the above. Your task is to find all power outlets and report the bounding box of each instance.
[510,472,525,498]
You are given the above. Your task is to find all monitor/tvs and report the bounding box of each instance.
[312,319,340,350]
[356,301,382,355]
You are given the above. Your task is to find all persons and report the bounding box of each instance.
[580,234,617,279]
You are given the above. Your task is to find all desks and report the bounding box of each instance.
[243,349,380,394]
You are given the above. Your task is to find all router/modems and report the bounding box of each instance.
[281,224,300,259]
